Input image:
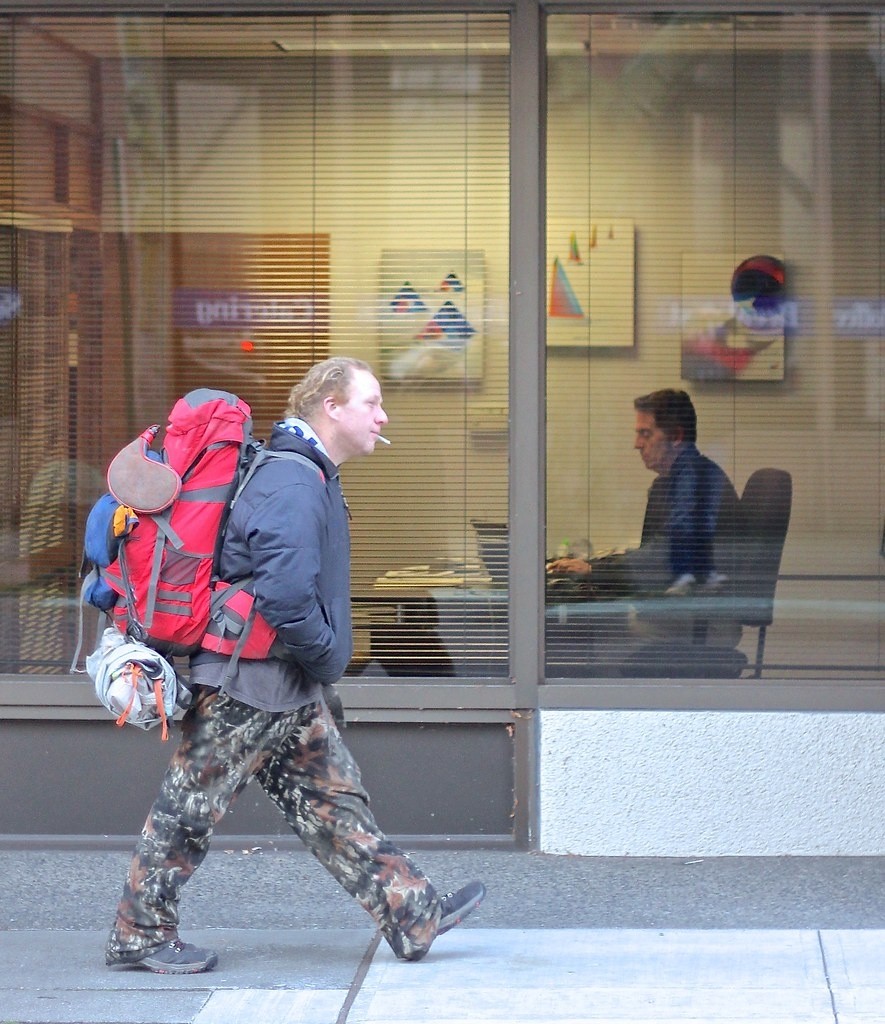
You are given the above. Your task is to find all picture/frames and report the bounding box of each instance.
[546,217,635,348]
[378,250,485,383]
[679,253,786,382]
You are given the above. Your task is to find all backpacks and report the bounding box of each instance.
[69,388,326,698]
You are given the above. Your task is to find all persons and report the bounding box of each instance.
[546,388,740,594]
[104,355,486,976]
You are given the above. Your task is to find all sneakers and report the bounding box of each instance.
[105,939,219,974]
[402,881,487,962]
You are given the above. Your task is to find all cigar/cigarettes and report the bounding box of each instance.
[378,435,390,445]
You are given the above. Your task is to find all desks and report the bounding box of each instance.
[351,563,606,682]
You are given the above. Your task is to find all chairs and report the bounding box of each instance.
[731,468,792,678]
